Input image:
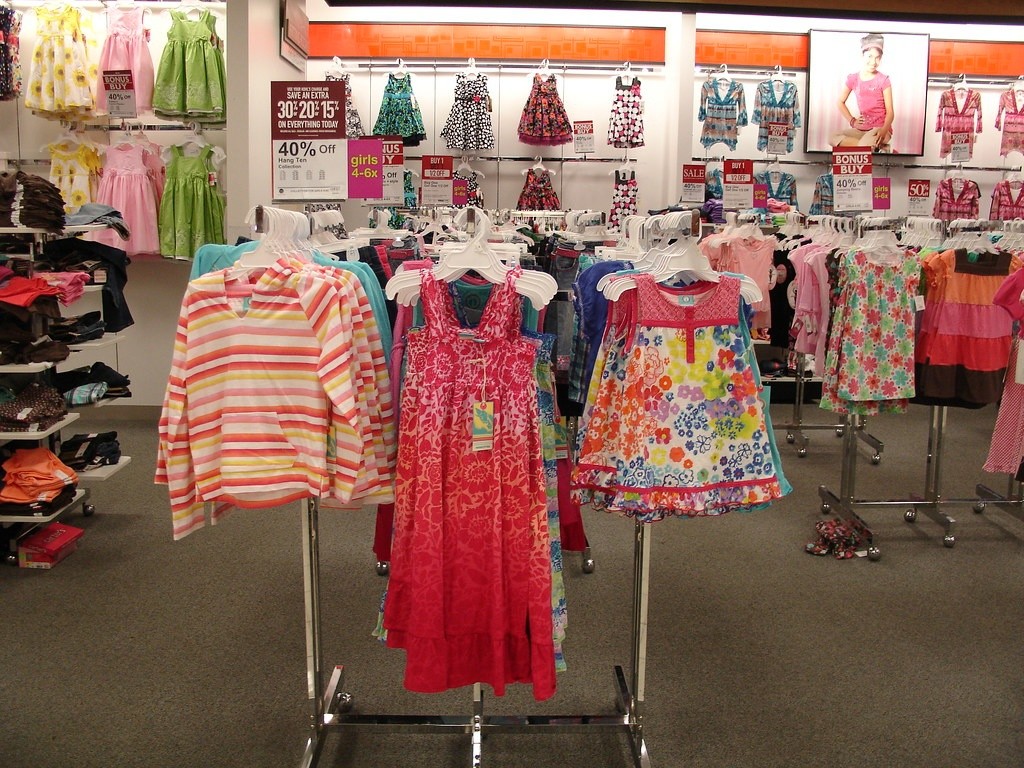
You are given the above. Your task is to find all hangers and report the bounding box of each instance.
[0,0,225,158]
[238,57,1024,311]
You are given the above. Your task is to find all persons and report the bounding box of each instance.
[827,32,895,155]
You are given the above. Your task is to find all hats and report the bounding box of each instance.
[860,33,884,54]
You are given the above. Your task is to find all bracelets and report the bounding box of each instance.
[850,117,856,126]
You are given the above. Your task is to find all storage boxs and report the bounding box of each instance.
[20,522,85,569]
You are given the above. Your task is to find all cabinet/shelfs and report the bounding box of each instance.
[0,224,133,559]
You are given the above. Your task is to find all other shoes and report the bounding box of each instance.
[878,143,893,153]
